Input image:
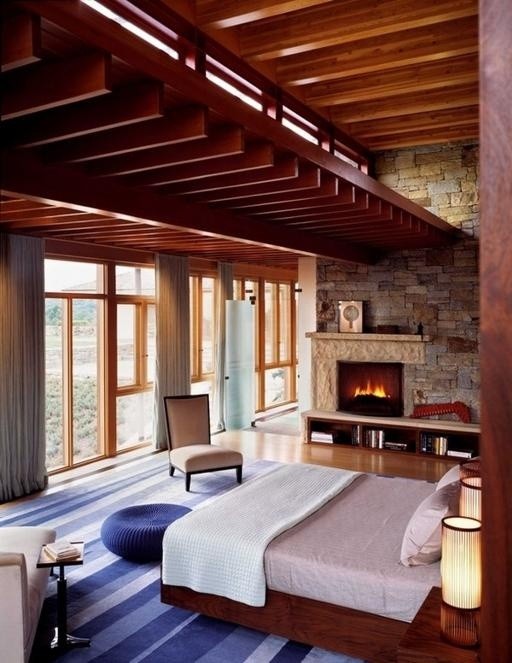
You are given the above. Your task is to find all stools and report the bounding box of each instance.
[100,504,193,563]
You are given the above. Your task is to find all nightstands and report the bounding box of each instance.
[394,586,479,661]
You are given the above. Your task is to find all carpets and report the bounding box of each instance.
[0,449,367,663]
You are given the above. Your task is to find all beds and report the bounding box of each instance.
[160,461,442,663]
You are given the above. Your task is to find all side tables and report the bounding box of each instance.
[37,541,90,653]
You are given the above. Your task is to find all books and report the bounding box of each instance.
[44,543,81,562]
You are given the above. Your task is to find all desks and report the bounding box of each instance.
[301,410,481,464]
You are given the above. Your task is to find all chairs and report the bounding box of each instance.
[0,526,56,662]
[164,394,243,492]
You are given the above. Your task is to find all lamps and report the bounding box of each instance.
[441,515,481,608]
[460,476,482,520]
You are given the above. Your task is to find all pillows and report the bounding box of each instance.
[400,464,461,568]
